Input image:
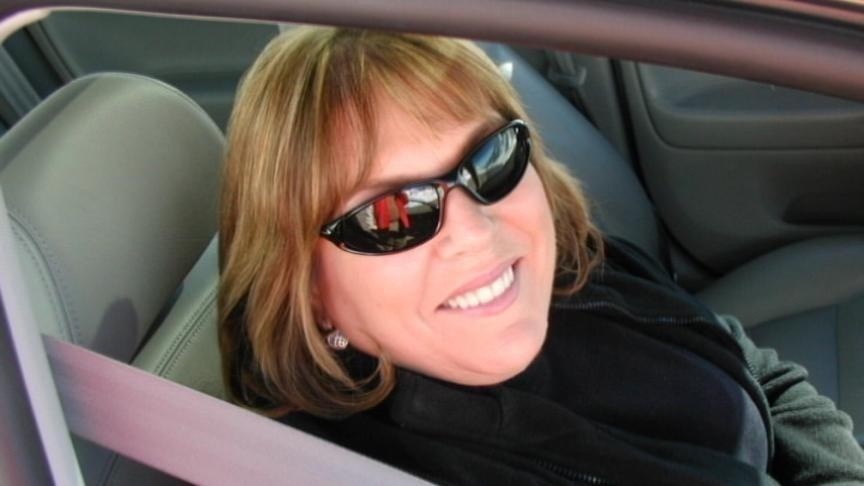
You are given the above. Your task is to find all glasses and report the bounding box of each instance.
[319,117,532,257]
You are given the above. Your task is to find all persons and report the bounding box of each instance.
[372,191,411,250]
[214,22,864,486]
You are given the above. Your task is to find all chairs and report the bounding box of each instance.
[474,43,864,447]
[0,70,230,486]
